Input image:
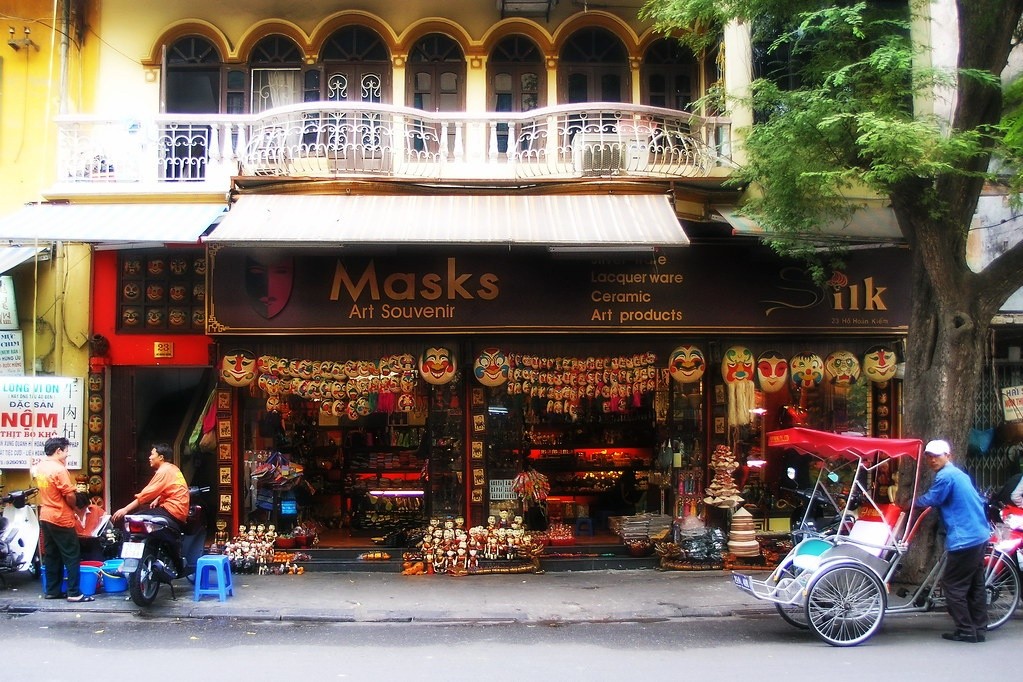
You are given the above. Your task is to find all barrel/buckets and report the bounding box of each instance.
[80,561,103,587]
[40,565,68,595]
[103,559,125,565]
[78,565,101,596]
[102,564,129,593]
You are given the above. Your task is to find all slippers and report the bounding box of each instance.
[67,593,95,602]
[45,592,67,599]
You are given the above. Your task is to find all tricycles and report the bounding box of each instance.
[731,426,1020,648]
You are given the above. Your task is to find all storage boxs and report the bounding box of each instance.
[534,538,551,546]
[551,536,576,546]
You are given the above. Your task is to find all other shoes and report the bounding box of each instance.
[941,630,986,643]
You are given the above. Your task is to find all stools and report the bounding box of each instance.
[576,518,595,536]
[194,555,234,602]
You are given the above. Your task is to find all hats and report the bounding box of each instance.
[924,439,951,455]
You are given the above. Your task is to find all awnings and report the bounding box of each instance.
[717,197,906,247]
[1,247,47,274]
[203,193,692,245]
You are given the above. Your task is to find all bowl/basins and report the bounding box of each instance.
[275,538,298,549]
[627,547,653,557]
[295,535,316,547]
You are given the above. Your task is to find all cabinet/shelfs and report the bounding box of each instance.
[339,411,431,530]
[522,395,661,495]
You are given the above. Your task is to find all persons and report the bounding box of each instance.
[31,437,96,602]
[111,443,190,558]
[901,439,1023,642]
[415,508,533,573]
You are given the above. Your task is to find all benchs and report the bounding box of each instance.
[793,504,901,576]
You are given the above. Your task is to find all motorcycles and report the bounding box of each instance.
[0,487,41,585]
[781,486,873,548]
[120,485,210,607]
[984,501,1023,601]
